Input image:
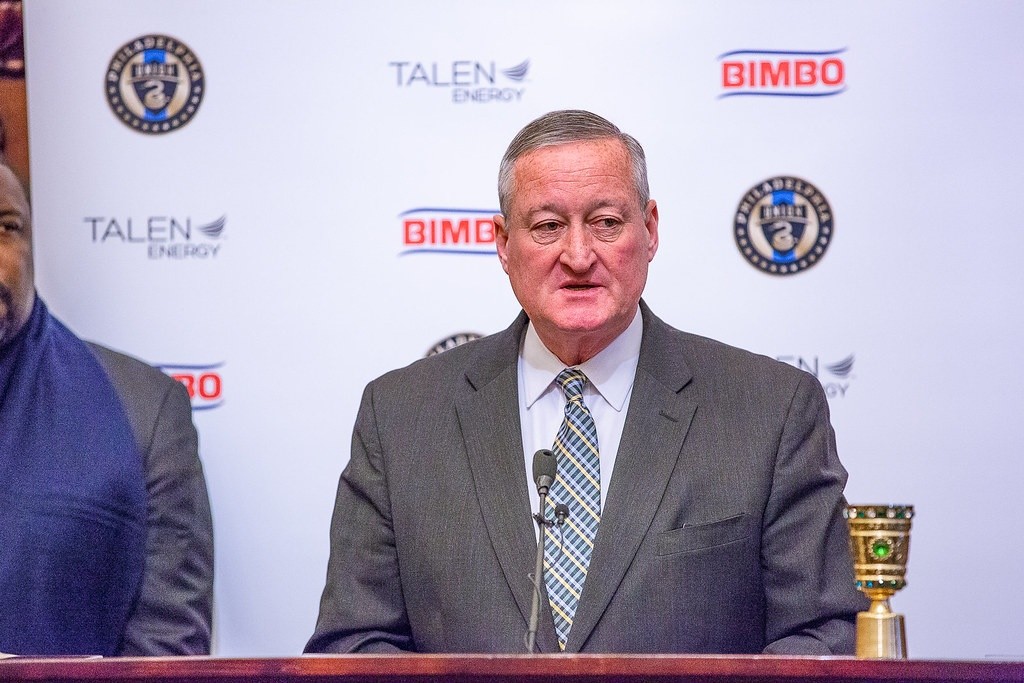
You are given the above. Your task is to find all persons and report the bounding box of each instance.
[0,159,215,657]
[298,110,872,657]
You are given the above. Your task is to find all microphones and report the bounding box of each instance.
[555,504,569,525]
[527,449,557,655]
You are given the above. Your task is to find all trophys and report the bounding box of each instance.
[844,504,915,660]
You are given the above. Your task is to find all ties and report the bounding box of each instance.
[542,369,600,651]
